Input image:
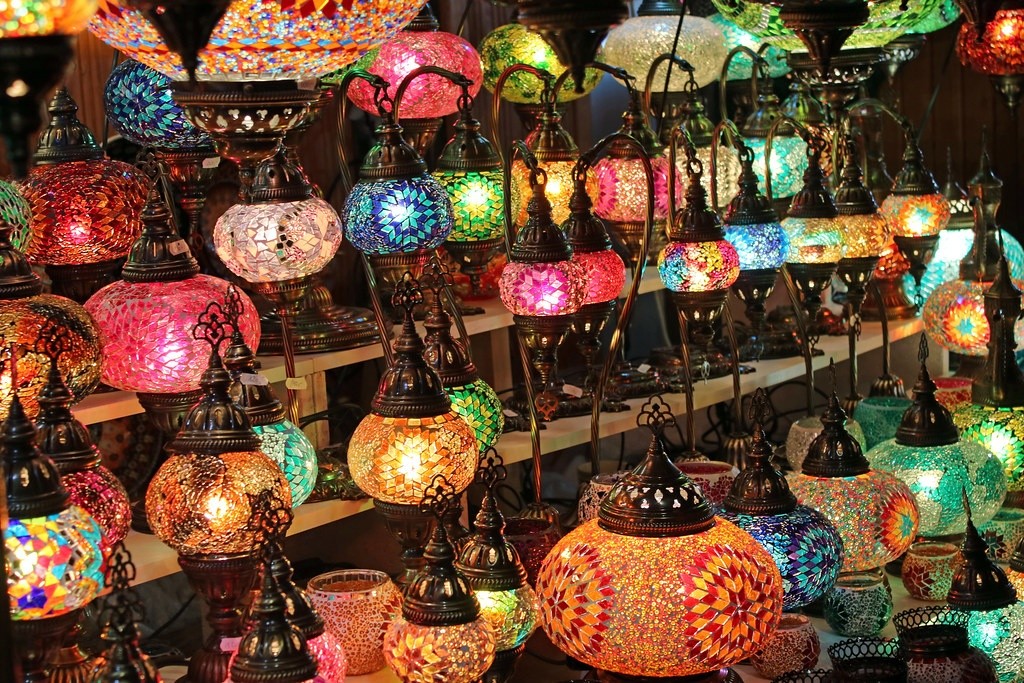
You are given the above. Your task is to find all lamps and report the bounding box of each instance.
[0,0,1024,683]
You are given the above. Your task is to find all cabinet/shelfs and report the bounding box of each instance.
[1,1,950,683]
